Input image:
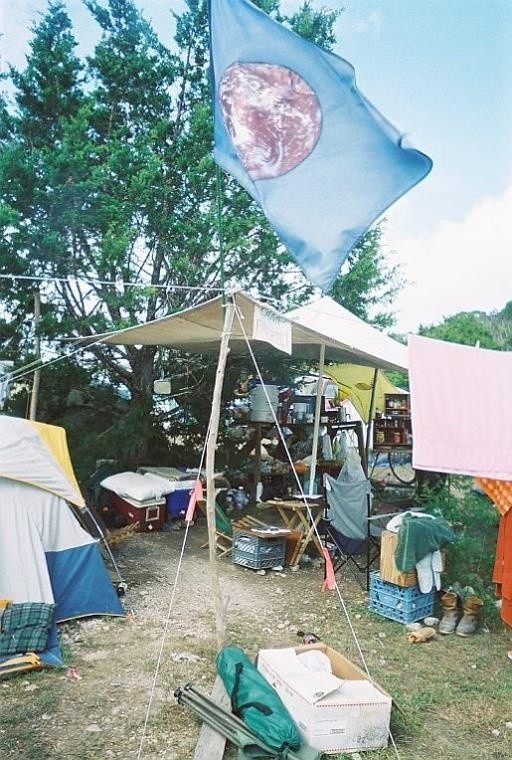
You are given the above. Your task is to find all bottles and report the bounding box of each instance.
[276,401,296,424]
[235,486,245,511]
[255,482,264,503]
[386,397,407,407]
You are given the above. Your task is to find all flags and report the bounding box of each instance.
[209,0,433,293]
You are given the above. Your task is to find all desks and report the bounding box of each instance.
[270,498,328,567]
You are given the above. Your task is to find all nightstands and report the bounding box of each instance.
[163,481,198,520]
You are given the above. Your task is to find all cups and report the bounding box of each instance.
[338,407,347,422]
[320,417,328,423]
[305,413,313,424]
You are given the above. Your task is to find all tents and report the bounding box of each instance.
[0,415,125,668]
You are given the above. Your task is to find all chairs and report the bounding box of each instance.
[193,497,235,558]
[320,472,385,592]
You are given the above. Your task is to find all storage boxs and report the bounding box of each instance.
[254,639,406,755]
[380,531,445,587]
[110,493,166,532]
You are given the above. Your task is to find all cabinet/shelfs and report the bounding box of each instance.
[371,392,412,451]
[240,419,368,508]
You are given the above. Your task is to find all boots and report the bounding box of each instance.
[439,592,460,635]
[456,596,484,636]
